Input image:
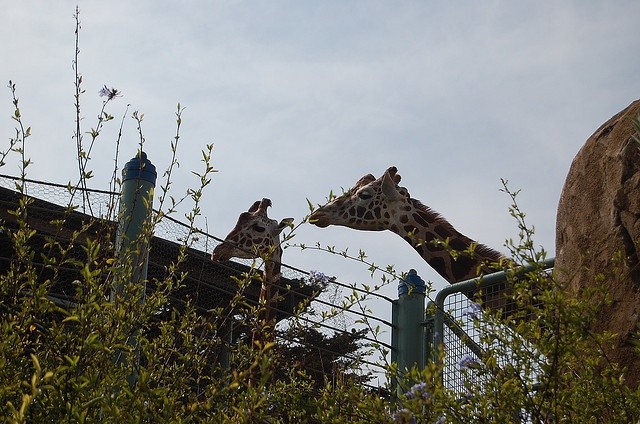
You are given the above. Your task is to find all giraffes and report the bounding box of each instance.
[308,165,554,358]
[211,198,295,402]
